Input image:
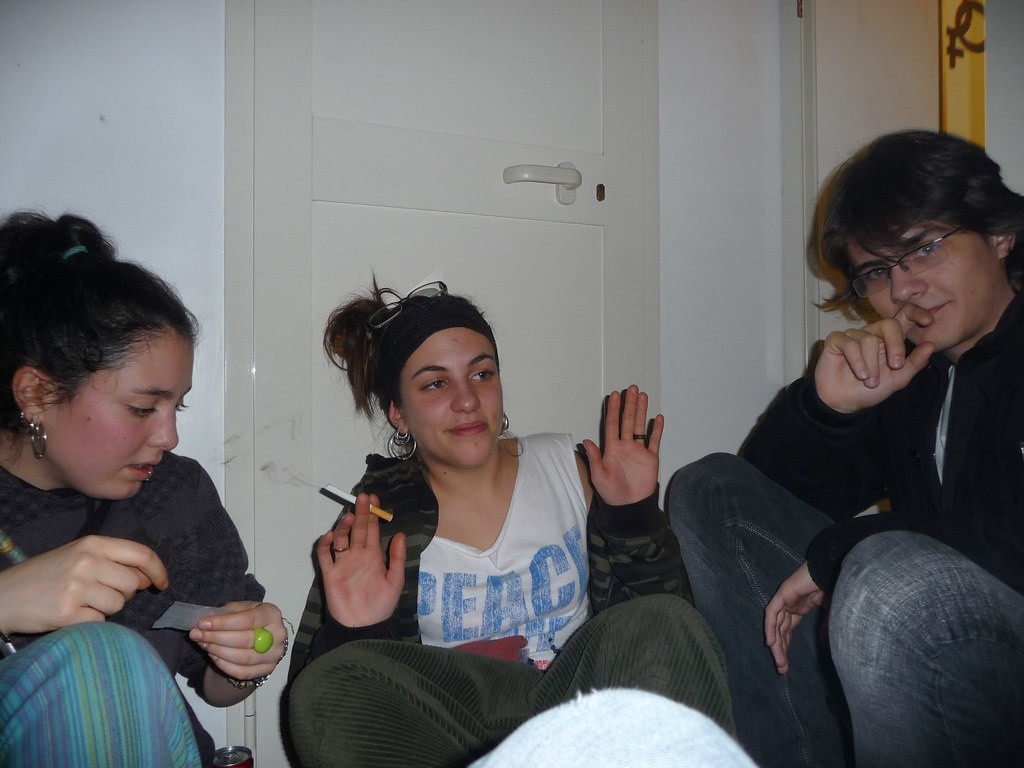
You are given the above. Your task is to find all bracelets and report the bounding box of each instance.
[226,617,294,690]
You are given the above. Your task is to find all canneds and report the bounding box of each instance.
[212,745,253,768]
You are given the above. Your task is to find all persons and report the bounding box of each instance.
[279,280,737,768]
[0,210,289,768]
[667,130,1024,768]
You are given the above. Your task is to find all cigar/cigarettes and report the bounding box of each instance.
[324,483,393,522]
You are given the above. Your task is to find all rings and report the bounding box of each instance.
[254,628,274,653]
[634,434,647,440]
[333,546,349,553]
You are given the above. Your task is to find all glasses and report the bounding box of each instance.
[851,227,961,299]
[368,280,448,328]
